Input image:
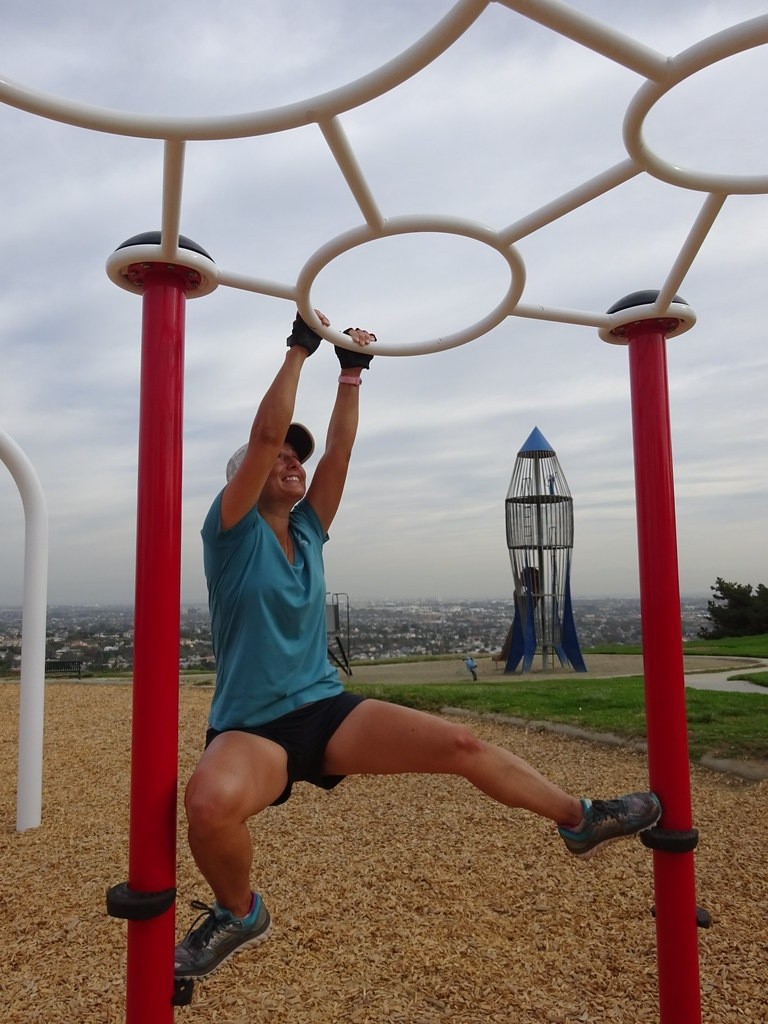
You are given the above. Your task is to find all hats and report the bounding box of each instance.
[227,423,315,486]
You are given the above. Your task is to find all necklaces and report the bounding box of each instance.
[287,535,289,558]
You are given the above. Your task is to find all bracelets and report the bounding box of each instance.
[338,376,362,385]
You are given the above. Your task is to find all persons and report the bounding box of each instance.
[175,311,663,982]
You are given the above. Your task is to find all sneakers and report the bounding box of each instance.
[557,791,662,861]
[174,890,274,981]
